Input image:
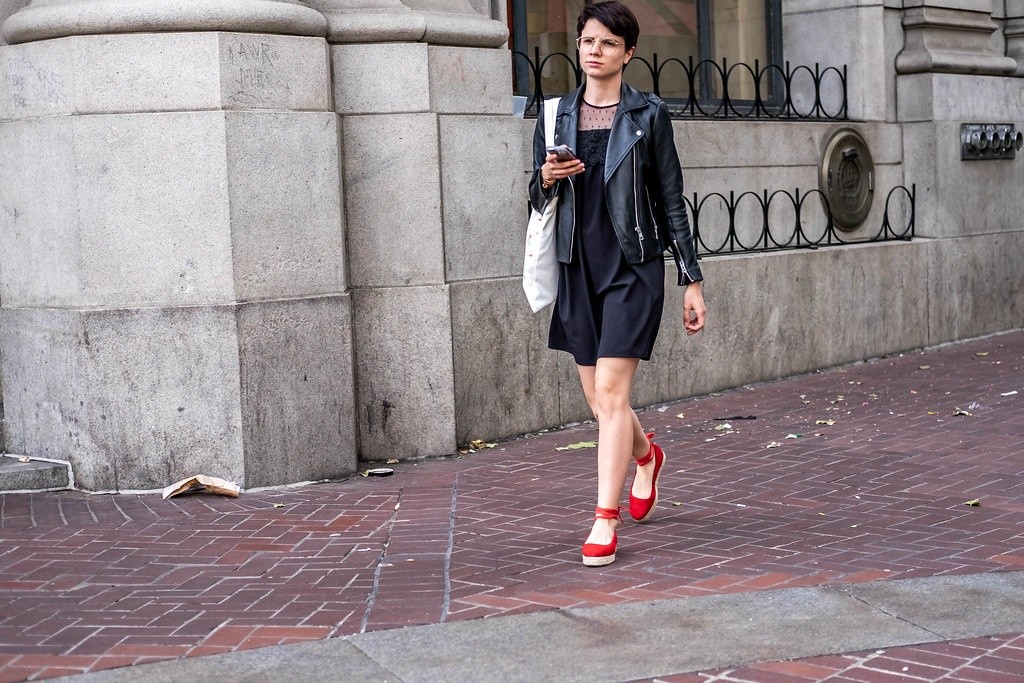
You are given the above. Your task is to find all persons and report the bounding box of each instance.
[528,0,707,566]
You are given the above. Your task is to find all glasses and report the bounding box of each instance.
[576,36,626,55]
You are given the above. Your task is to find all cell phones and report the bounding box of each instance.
[546,145,578,162]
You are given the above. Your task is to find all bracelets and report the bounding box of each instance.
[542,176,556,188]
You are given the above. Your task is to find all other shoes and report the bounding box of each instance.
[582,506,630,566]
[630,432,665,523]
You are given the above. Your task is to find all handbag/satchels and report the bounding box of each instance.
[523,97,559,313]
